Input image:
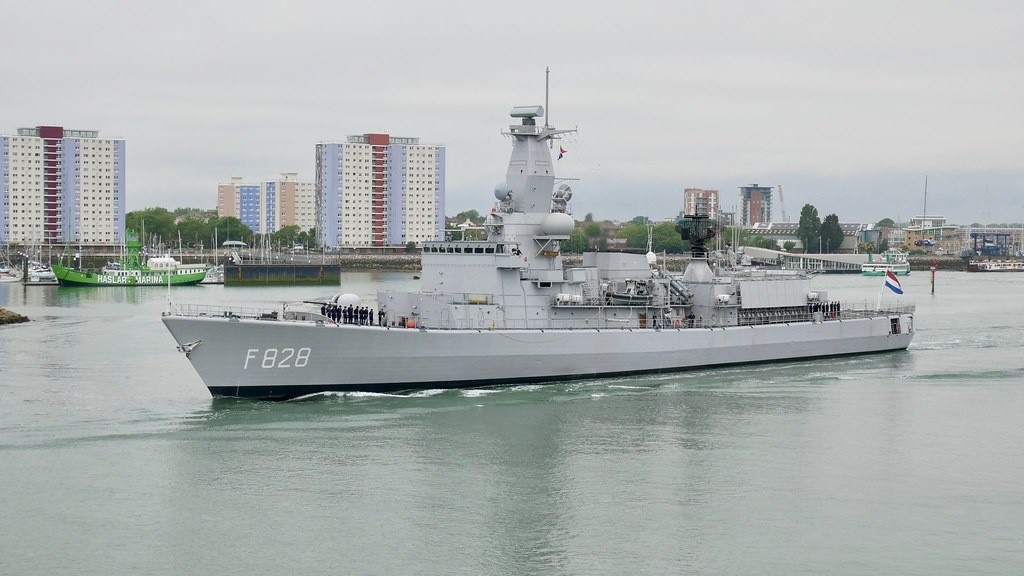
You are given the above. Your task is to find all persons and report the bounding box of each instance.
[378,309,386,326]
[652,312,657,328]
[321,303,374,327]
[809,301,840,318]
[688,311,695,327]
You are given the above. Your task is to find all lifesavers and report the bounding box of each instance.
[831,311,836,318]
[674,319,680,327]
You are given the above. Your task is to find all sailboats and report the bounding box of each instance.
[50,206,207,288]
[663,203,815,284]
[0,220,340,286]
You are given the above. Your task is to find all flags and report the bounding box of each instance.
[885,270,903,295]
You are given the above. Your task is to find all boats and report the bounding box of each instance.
[160,65,917,405]
[862,246,911,276]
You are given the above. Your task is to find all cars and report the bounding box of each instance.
[914,237,936,247]
